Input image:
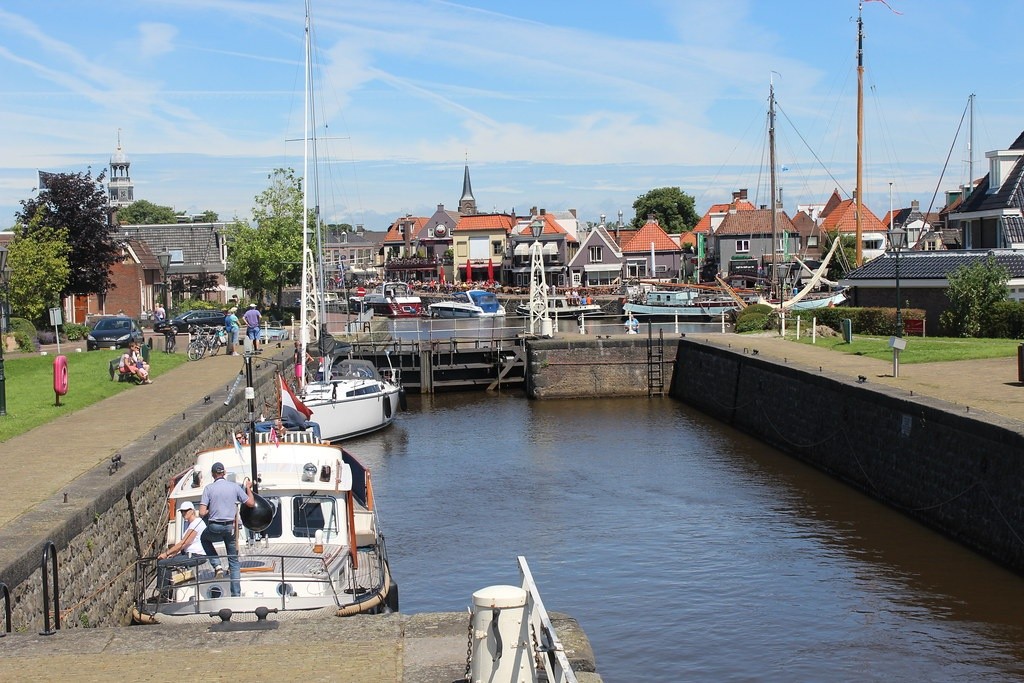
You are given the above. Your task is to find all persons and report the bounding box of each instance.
[199,462,255,597]
[119,342,153,384]
[225,307,241,356]
[624,315,640,334]
[364,277,499,292]
[147,501,207,603]
[243,303,262,354]
[564,288,591,305]
[155,303,166,320]
[293,341,314,393]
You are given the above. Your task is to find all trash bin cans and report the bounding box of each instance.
[1017,342,1024,381]
[141,342,151,364]
[840,319,852,341]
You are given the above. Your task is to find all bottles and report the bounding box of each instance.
[302,461,317,482]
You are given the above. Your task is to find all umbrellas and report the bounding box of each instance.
[466,259,472,282]
[488,259,494,283]
[440,264,444,284]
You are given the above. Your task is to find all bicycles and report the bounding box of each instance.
[165,326,176,355]
[188,324,222,361]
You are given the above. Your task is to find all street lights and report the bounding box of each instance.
[0,246,9,416]
[1,264,13,333]
[889,182,893,230]
[528,219,552,338]
[299,225,320,344]
[157,245,172,326]
[885,219,907,338]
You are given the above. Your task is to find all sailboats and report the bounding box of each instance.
[621,81,854,323]
[262,0,405,445]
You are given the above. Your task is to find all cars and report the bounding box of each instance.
[153,310,226,336]
[259,321,289,345]
[87,316,145,352]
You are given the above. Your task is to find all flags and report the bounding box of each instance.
[280,377,313,427]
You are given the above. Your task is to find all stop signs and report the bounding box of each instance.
[356,288,366,296]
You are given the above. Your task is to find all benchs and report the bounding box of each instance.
[109,357,133,382]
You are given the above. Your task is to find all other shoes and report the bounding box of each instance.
[148,592,172,603]
[233,352,239,356]
[213,568,223,579]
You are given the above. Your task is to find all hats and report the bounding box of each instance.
[250,304,257,308]
[212,462,224,473]
[177,502,194,512]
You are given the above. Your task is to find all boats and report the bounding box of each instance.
[513,290,606,320]
[296,282,507,319]
[132,354,401,624]
